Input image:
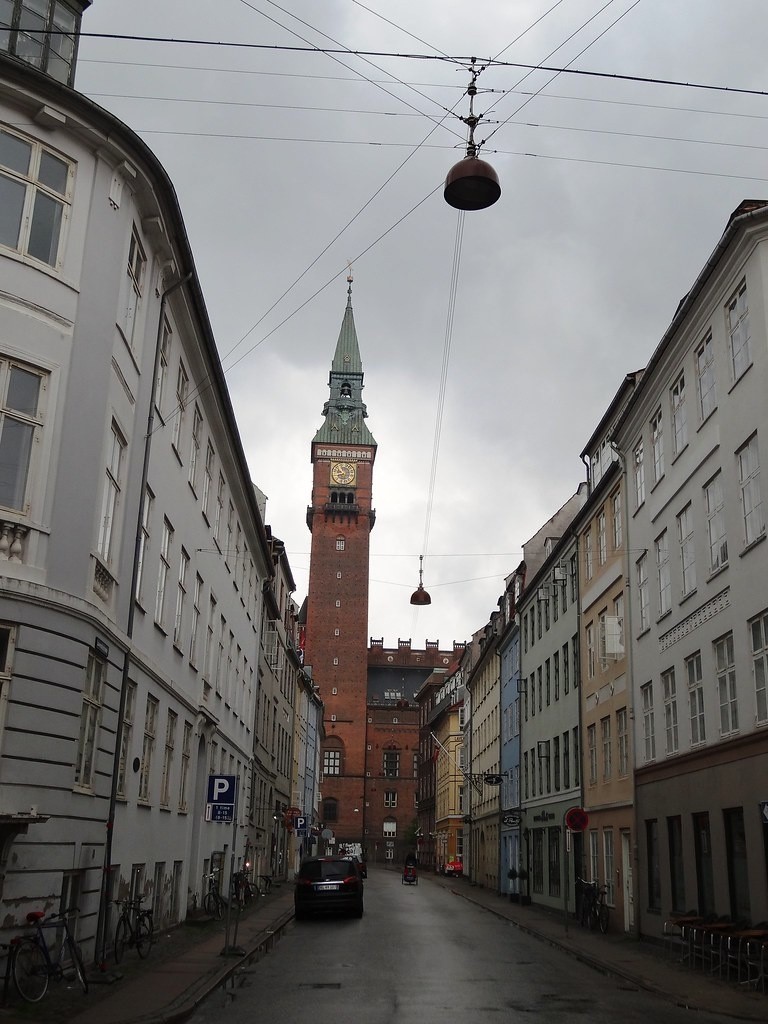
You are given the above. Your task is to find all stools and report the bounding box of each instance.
[666,915,768,995]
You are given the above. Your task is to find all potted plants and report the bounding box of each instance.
[507,867,519,903]
[517,868,531,906]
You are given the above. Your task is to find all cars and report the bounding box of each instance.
[440,860,463,877]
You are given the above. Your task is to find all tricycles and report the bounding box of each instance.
[398,864,419,884]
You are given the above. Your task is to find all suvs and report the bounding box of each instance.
[290,855,362,918]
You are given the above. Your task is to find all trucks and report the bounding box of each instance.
[338,849,368,877]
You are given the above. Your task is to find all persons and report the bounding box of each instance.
[405,850,417,869]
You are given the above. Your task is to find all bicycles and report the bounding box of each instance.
[575,876,610,933]
[203,867,227,918]
[110,896,156,963]
[12,906,89,1003]
[233,864,274,909]
